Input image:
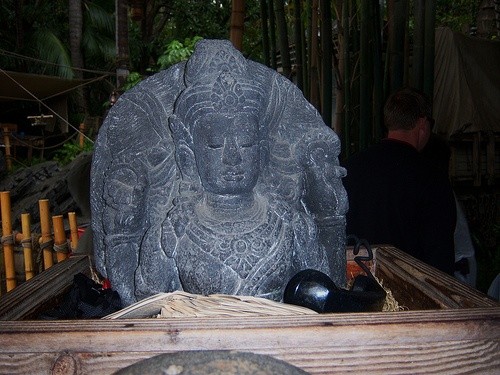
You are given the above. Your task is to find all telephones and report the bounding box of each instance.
[283,269,386,314]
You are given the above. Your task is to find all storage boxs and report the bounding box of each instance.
[0,242,500,375]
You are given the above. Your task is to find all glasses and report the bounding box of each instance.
[417,114,435,131]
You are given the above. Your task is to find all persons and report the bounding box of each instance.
[346,89,458,276]
[89,38,349,301]
